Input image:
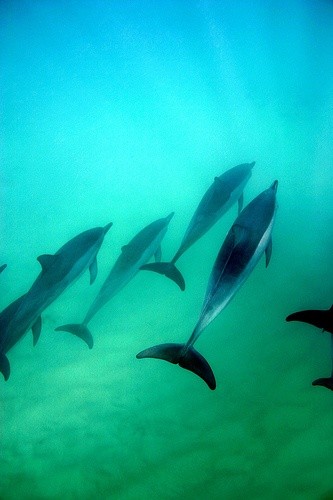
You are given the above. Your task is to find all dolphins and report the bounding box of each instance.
[135,179,279,391]
[0,222,113,380]
[54,210,174,349]
[139,160,256,291]
[0,293,42,382]
